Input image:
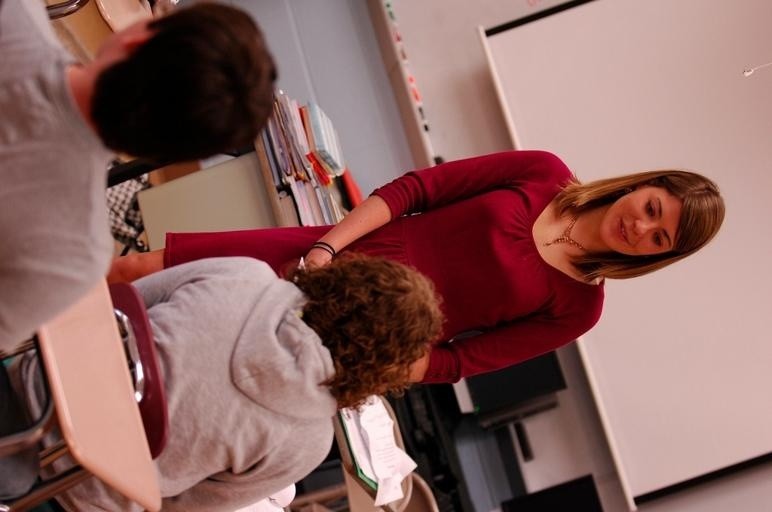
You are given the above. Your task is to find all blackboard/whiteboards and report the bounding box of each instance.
[367,0,772,512]
